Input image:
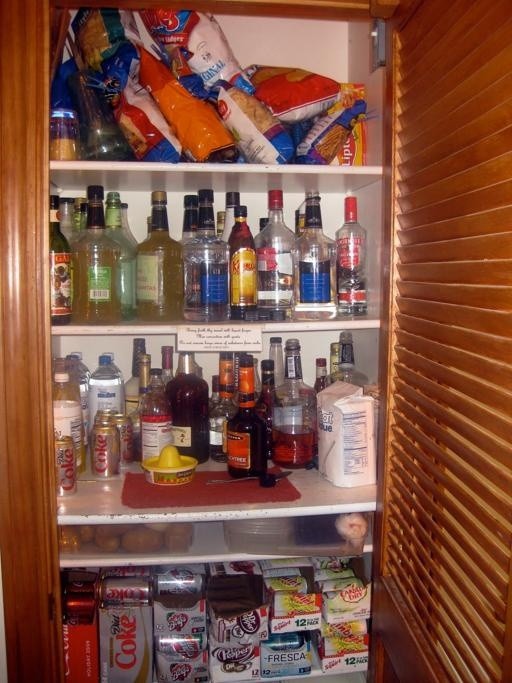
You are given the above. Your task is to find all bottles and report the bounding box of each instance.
[55,436,77,496]
[94,409,113,423]
[183,189,230,320]
[168,350,210,464]
[68,70,132,161]
[49,109,80,160]
[153,632,207,656]
[262,633,304,653]
[255,359,280,458]
[124,338,148,414]
[271,339,318,468]
[78,202,90,240]
[141,369,172,461]
[316,579,364,594]
[291,192,337,320]
[318,618,367,637]
[336,197,368,315]
[63,617,95,626]
[209,351,237,461]
[292,213,307,240]
[210,376,222,414]
[217,212,227,240]
[135,191,183,322]
[160,347,173,386]
[155,574,205,596]
[314,557,355,569]
[89,355,121,433]
[63,580,96,594]
[52,373,86,478]
[210,643,255,662]
[48,195,73,324]
[147,217,153,237]
[250,358,261,405]
[269,337,284,389]
[91,421,120,478]
[56,197,79,246]
[96,576,153,606]
[121,204,138,245]
[227,355,266,478]
[55,355,88,448]
[267,575,309,594]
[315,567,356,580]
[180,195,199,246]
[72,195,88,230]
[104,192,136,319]
[64,592,96,616]
[259,218,269,231]
[101,568,154,578]
[253,190,295,319]
[326,333,370,395]
[103,353,123,414]
[72,184,121,325]
[70,351,93,377]
[227,207,257,319]
[110,414,134,464]
[128,354,154,461]
[221,192,242,246]
[314,358,328,393]
[331,342,341,373]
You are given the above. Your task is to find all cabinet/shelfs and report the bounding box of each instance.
[28,0,397,683]
